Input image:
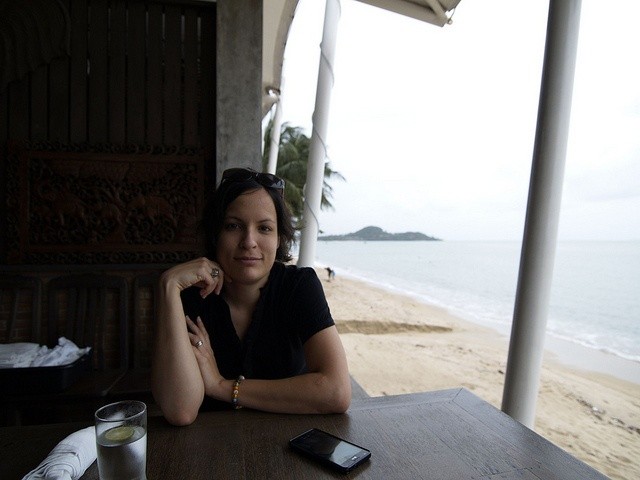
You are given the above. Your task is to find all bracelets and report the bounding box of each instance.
[233,376,245,410]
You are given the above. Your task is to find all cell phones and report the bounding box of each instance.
[289,427,371,474]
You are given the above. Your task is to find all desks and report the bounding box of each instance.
[0,388,612,480]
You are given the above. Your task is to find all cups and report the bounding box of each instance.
[95,400,148,480]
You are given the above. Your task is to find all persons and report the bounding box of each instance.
[146,166,351,427]
[322,266,334,281]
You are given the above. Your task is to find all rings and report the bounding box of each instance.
[193,341,203,348]
[211,268,219,278]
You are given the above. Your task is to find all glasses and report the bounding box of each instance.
[219,167,285,201]
